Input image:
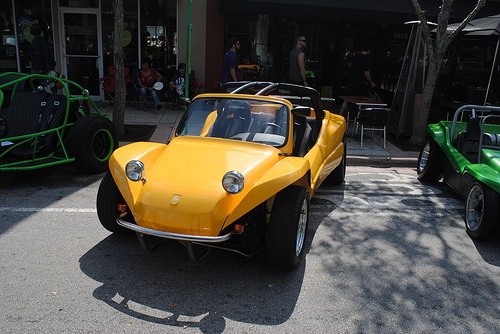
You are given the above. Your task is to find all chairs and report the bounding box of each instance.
[206,100,254,139]
[264,105,313,157]
[344,106,391,149]
[454,117,489,164]
[95,66,199,111]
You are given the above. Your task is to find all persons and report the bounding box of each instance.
[288,36,308,87]
[170,63,186,97]
[223,38,240,82]
[45,60,57,94]
[340,45,376,115]
[136,61,163,110]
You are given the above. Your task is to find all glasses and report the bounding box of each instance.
[299,40,307,42]
[235,43,240,46]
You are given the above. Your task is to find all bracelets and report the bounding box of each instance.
[303,77,306,81]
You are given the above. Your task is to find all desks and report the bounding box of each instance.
[339,96,387,143]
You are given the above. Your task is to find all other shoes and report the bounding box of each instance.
[144,107,149,111]
[157,104,161,111]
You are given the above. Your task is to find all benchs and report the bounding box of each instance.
[0,92,67,164]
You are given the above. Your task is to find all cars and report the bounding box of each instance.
[94,79,350,273]
[416,103,500,240]
[0,71,119,177]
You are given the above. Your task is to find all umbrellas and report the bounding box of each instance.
[431,14,500,115]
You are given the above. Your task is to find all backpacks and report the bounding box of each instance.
[50,71,65,89]
